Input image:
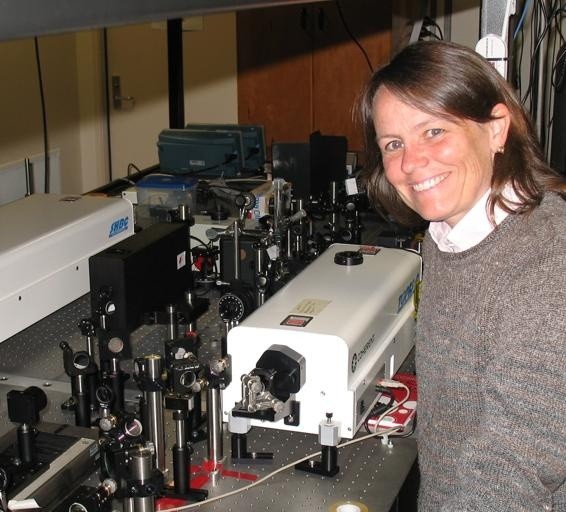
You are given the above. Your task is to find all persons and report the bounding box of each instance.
[353,39,566,511]
